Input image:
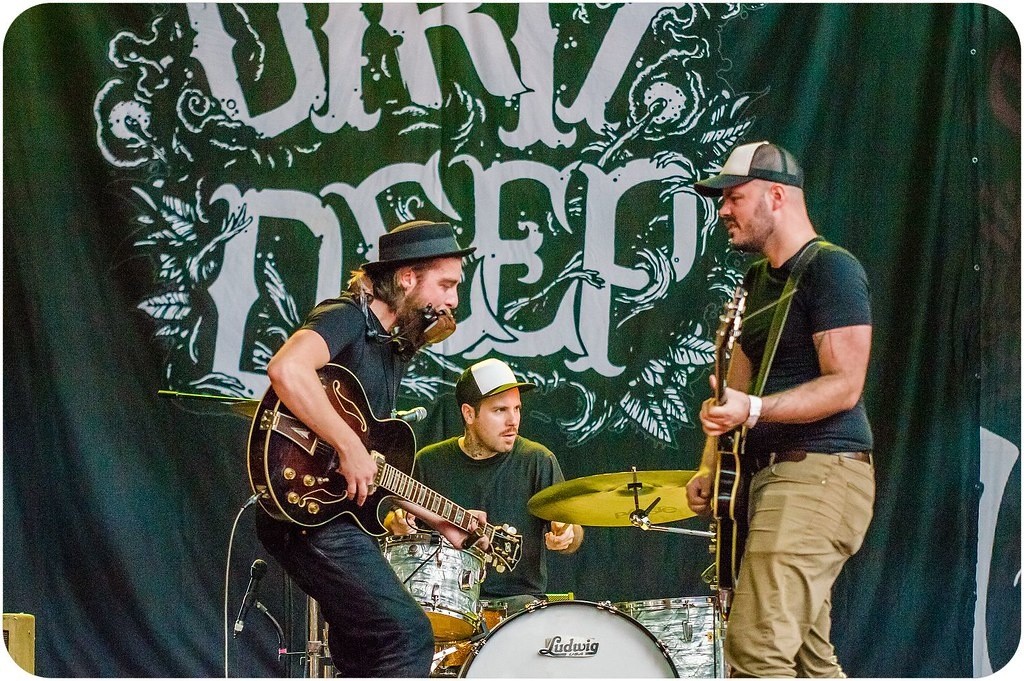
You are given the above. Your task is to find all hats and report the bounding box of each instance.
[693,141,807,198]
[362,221,477,273]
[456,357,537,404]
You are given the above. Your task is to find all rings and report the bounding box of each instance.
[367,481,374,485]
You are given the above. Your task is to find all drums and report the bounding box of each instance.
[374,531,487,644]
[458,601,678,676]
[429,603,508,678]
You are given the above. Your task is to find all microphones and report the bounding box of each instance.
[232,559,267,637]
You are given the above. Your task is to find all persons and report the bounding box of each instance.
[684,138,877,680]
[257,218,478,678]
[382,357,585,622]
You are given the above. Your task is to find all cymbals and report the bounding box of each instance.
[529,470,714,527]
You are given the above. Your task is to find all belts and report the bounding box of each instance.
[749,449,874,475]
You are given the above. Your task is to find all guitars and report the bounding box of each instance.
[249,366,522,576]
[717,289,749,589]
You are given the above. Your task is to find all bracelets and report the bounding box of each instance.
[743,393,763,429]
